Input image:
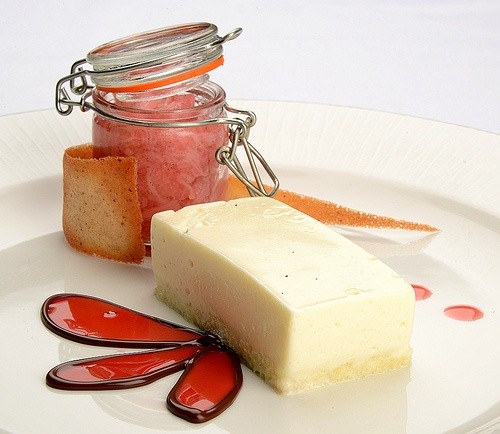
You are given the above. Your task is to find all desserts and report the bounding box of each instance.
[149,196,415,394]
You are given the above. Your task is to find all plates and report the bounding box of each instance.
[0,105,500,434]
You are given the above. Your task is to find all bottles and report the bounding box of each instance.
[55,22,280,257]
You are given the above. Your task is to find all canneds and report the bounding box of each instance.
[56,21,279,256]
